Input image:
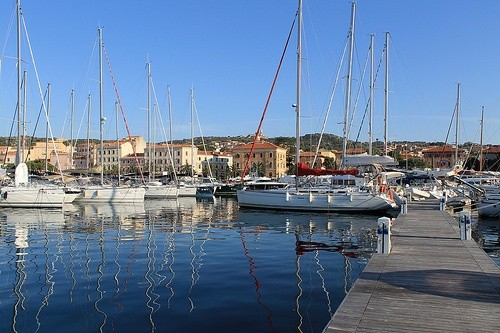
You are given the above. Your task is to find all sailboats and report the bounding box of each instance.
[397,82,500,218]
[0,0,145,208]
[236,0,408,218]
[122,53,272,199]
